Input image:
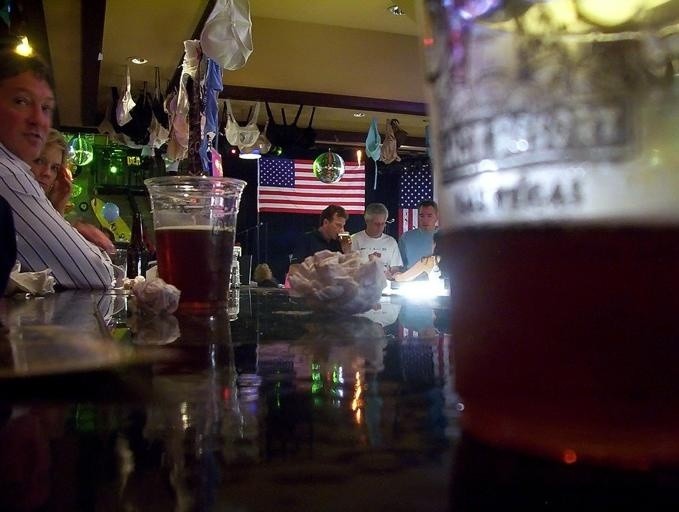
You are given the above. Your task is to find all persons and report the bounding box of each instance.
[32,124,74,223]
[398,200,440,269]
[350,293,457,348]
[350,202,404,280]
[298,203,353,274]
[386,226,449,283]
[0,39,115,289]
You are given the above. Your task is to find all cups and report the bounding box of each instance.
[337,230,352,252]
[143,175,249,321]
[415,0,679,473]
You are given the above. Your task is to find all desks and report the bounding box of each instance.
[1,284,677,512]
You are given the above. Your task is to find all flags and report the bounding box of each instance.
[258,156,366,218]
[399,164,436,241]
